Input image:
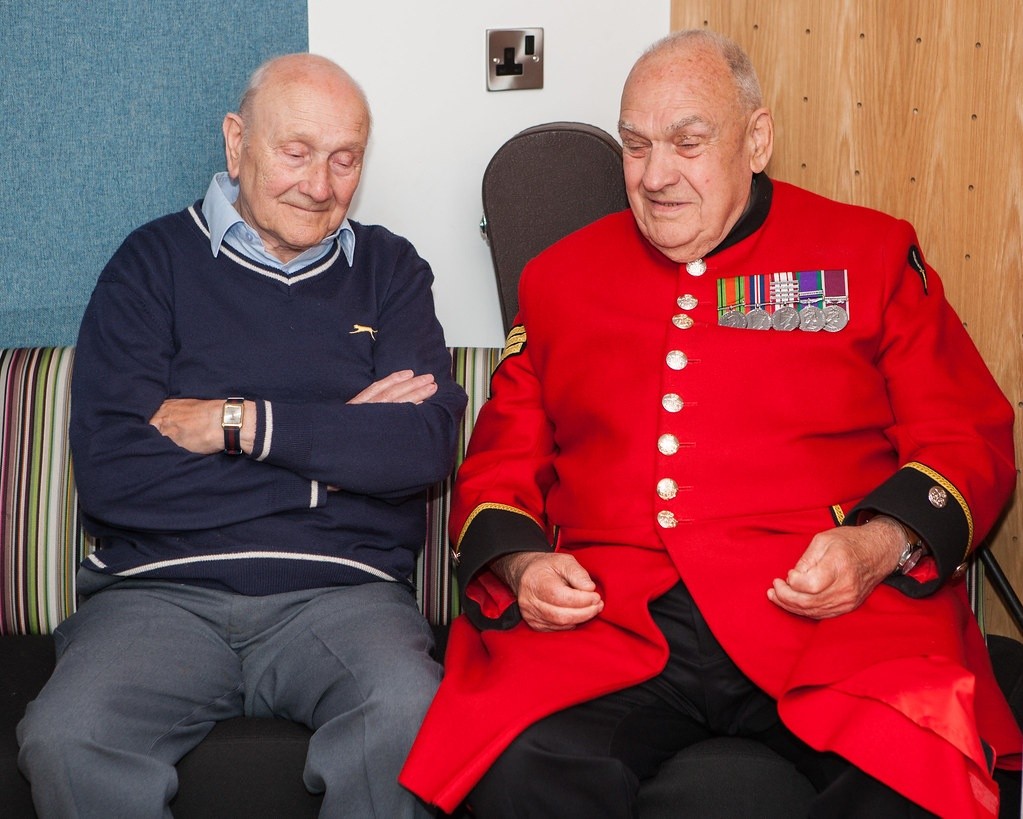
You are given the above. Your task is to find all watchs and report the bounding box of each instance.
[221,396,244,458]
[861,514,927,577]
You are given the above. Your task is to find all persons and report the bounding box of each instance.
[17,53,473,819]
[395,31,1022,819]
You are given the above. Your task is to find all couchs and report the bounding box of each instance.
[0,346,1023,819]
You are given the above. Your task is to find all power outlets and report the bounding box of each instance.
[486,27,544,91]
